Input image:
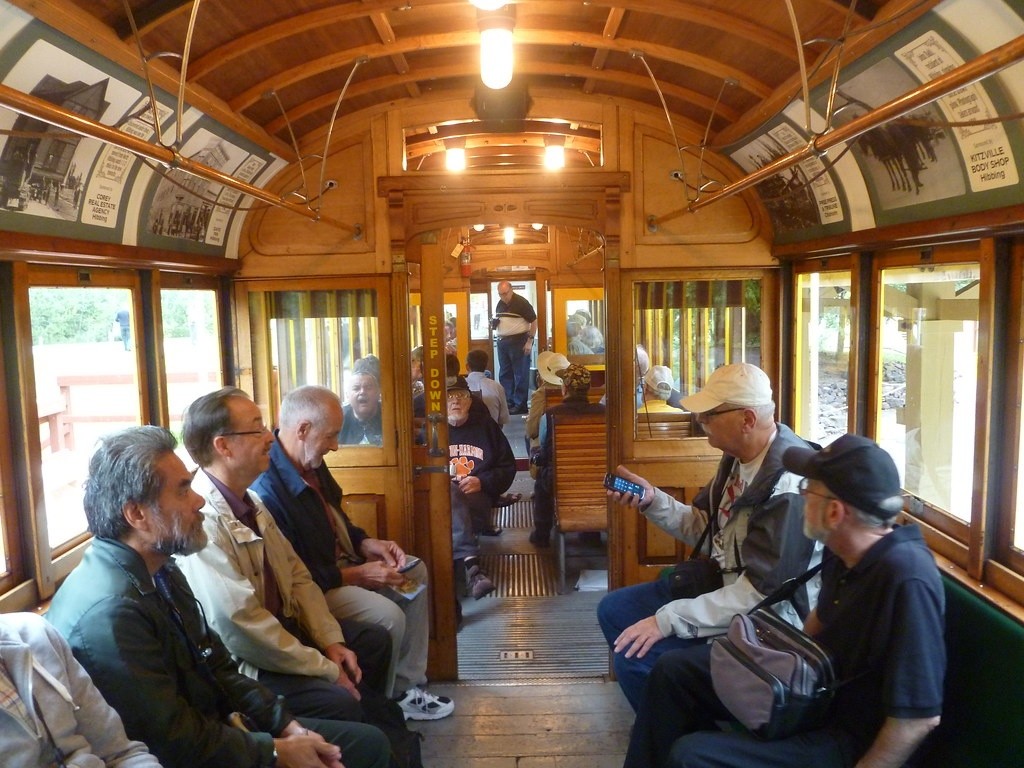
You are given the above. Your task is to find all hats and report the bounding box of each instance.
[555,365,591,388]
[537,351,571,386]
[679,363,772,413]
[568,314,587,327]
[645,365,674,393]
[445,376,469,392]
[782,433,904,519]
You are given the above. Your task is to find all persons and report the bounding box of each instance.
[115,303,132,352]
[445,310,457,353]
[44,425,348,768]
[0,611,166,768]
[524,341,686,550]
[169,384,397,768]
[408,341,518,600]
[620,432,952,768]
[247,383,455,722]
[333,353,383,444]
[596,361,828,719]
[489,280,537,415]
[474,301,481,330]
[566,308,605,353]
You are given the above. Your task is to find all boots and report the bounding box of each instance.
[530,508,554,547]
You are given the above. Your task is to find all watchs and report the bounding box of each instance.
[528,335,534,340]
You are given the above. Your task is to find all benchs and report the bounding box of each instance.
[535,365,724,596]
[915,578,1024,768]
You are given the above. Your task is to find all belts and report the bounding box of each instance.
[497,332,527,340]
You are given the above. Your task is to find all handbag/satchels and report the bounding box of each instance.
[356,702,424,768]
[709,606,840,741]
[668,556,726,600]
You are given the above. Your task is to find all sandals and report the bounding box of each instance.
[491,492,522,507]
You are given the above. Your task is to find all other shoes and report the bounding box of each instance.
[506,402,528,414]
[481,526,502,536]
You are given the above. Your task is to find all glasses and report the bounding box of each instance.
[211,422,267,445]
[498,291,510,297]
[560,379,564,383]
[640,381,648,389]
[797,477,848,515]
[174,596,213,665]
[446,392,469,400]
[705,407,757,419]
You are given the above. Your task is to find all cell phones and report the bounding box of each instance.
[396,558,422,573]
[603,473,645,500]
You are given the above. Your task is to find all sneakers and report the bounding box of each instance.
[391,686,454,721]
[465,563,496,600]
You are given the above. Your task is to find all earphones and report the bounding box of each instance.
[201,647,213,657]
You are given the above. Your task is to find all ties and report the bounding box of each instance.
[154,571,216,684]
